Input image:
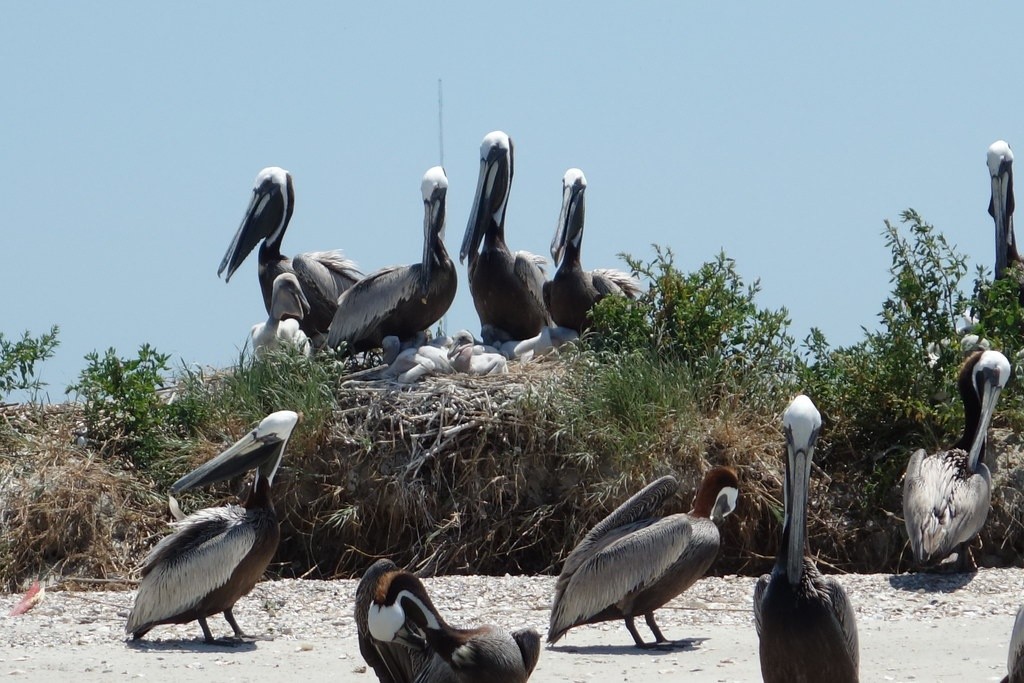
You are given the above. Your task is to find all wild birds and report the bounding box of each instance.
[217,130,636,382]
[1000,605,1024,683]
[546,466,739,648]
[987,141,1024,281]
[894,348,1010,575]
[354,557,540,683]
[120,410,298,644]
[753,395,860,683]
